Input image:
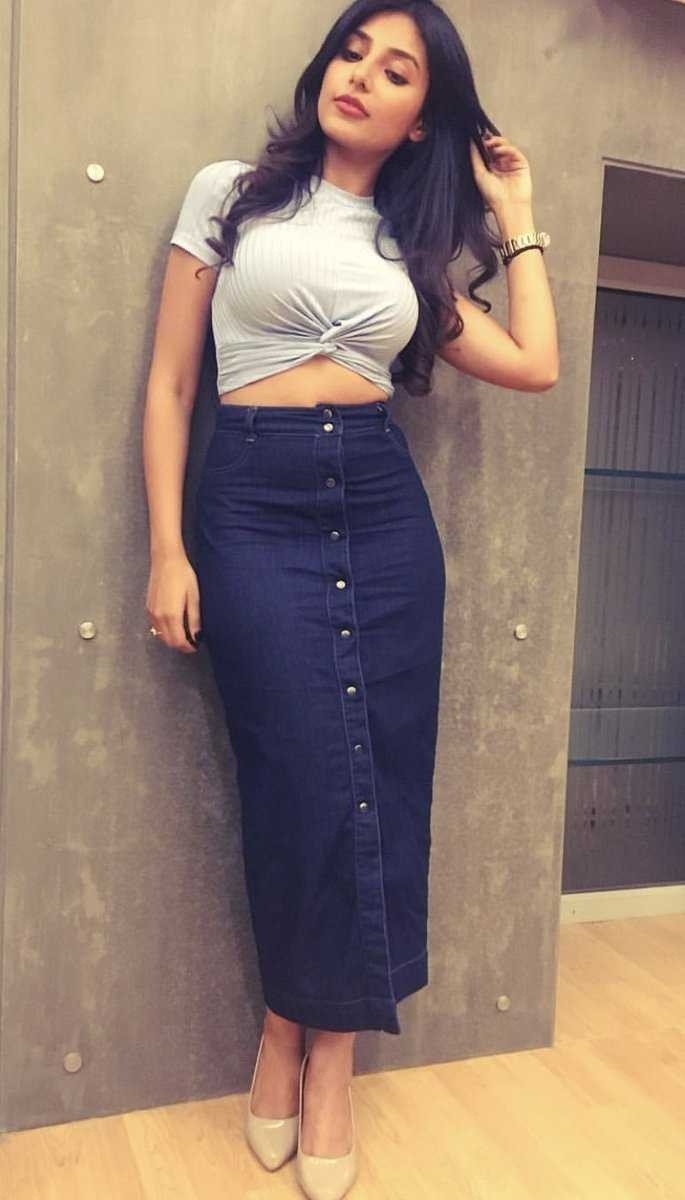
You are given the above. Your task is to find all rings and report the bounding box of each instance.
[149,627,162,638]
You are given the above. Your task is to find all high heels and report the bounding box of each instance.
[246,1034,299,1172]
[297,1055,355,1200]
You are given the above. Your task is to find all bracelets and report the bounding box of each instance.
[498,232,550,264]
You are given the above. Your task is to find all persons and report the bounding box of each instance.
[138,1,558,1200]
